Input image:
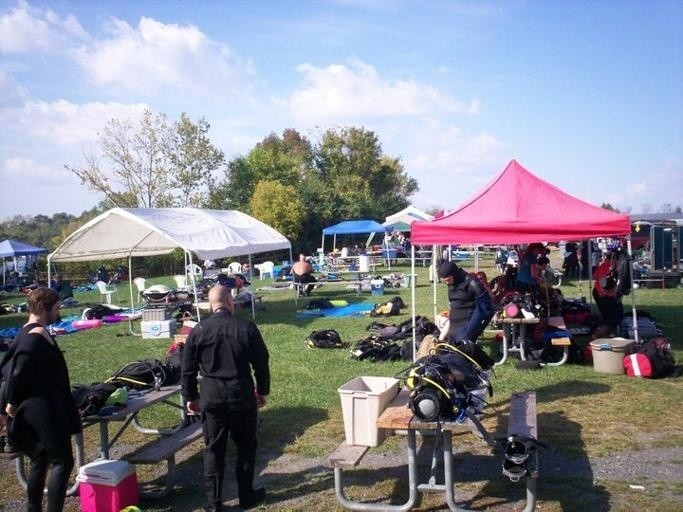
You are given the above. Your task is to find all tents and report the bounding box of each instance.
[47,209,298,328]
[1,239,48,289]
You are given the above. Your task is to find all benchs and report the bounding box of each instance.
[124,422,206,498]
[123,315,142,337]
[294,250,473,296]
[329,440,372,512]
[507,389,541,512]
[546,316,571,366]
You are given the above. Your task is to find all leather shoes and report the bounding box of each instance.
[239,486,265,509]
[203,499,221,512]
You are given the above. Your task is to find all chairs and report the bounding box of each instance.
[96,275,188,306]
[186,260,273,283]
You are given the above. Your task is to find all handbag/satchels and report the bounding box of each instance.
[623,340,674,379]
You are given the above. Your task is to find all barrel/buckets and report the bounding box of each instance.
[359,254,370,272]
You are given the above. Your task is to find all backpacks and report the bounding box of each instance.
[600,245,631,299]
[303,329,350,351]
[70,342,185,418]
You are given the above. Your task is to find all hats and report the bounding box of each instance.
[438,262,460,286]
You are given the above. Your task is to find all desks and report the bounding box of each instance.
[369,375,496,511]
[493,314,539,366]
[83,376,203,498]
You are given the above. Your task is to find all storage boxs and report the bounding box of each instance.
[140,308,177,340]
[589,338,635,375]
[75,458,139,512]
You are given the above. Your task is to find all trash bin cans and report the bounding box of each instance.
[406,274,418,288]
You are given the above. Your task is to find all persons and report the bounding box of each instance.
[1,286,82,512]
[290,253,318,297]
[342,244,363,263]
[438,238,631,347]
[181,283,270,511]
[51,274,62,291]
[92,267,126,284]
